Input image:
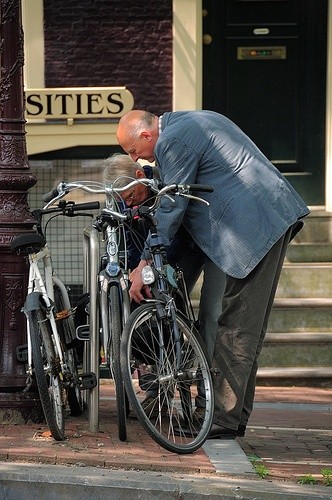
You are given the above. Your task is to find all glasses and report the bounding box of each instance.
[123,187,135,201]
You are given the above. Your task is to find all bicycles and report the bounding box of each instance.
[93,183,222,454]
[8,199,101,442]
[43,175,178,441]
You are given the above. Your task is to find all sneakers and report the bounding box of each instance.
[194,407,206,419]
[127,396,168,420]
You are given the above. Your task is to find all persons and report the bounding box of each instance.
[102,155,203,420]
[115,108,312,439]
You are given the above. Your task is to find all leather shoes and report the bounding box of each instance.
[171,417,246,441]
